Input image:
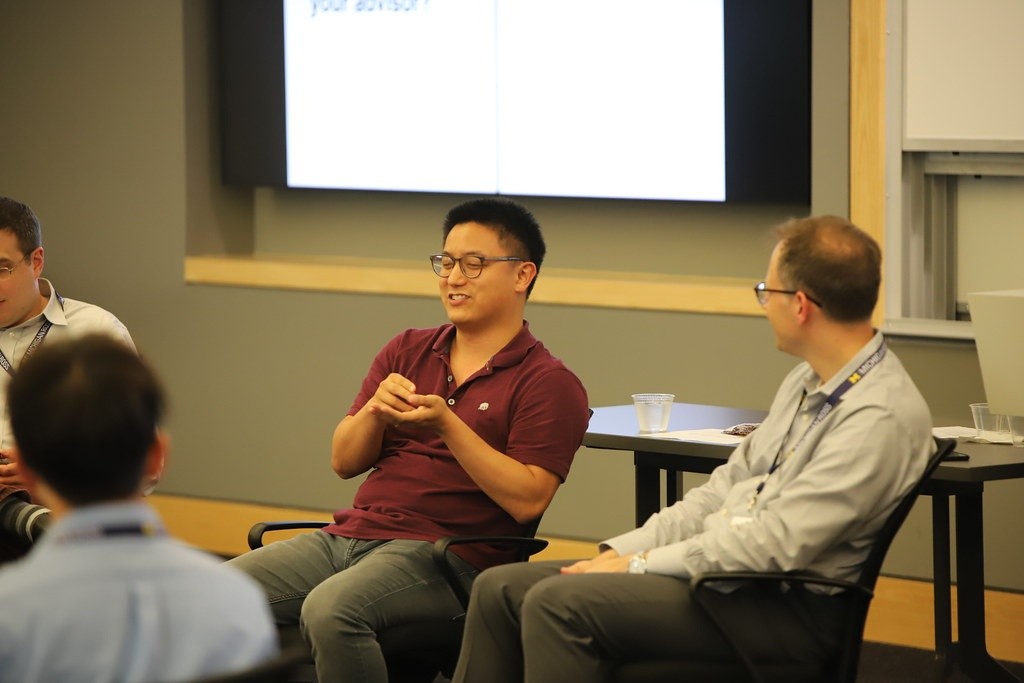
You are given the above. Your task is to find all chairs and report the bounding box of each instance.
[245,516,549,681]
[611,436,956,682]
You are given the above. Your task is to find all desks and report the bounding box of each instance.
[584,399,1023,682]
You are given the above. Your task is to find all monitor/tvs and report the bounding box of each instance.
[215,1,812,209]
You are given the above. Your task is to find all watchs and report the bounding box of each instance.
[628,550,648,574]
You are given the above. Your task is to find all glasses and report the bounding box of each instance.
[0,250,32,280]
[429,252,526,279]
[754,281,822,311]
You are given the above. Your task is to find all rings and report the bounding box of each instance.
[395,424,398,427]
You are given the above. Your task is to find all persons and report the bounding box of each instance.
[450,215,933,683]
[1,196,141,564]
[225,197,589,683]
[0,333,279,683]
